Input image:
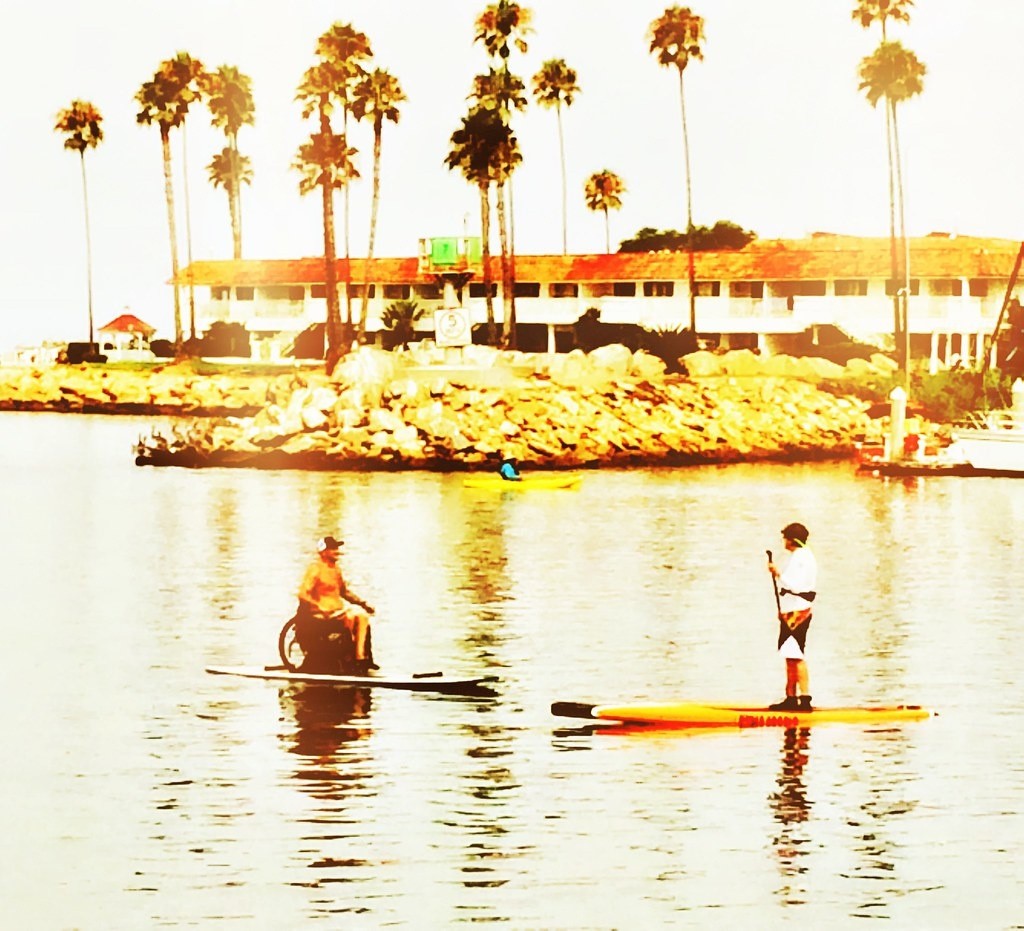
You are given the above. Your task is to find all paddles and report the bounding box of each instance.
[763,549,783,619]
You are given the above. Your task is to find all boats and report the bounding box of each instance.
[952,412,1024,473]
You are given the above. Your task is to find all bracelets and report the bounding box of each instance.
[360,601,366,606]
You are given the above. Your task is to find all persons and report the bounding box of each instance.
[769,524,816,712]
[299,537,379,674]
[937,432,963,468]
[501,453,522,481]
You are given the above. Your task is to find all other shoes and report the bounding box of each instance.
[769,695,813,711]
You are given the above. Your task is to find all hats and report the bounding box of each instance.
[781,523,809,547]
[317,537,344,553]
